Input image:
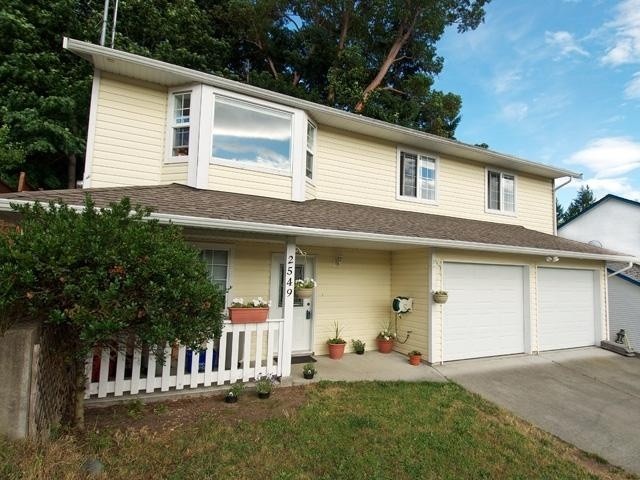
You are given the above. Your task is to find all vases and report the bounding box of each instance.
[378,338,394,352]
[229,307,269,324]
[298,290,313,299]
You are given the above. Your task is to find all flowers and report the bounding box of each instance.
[294,276,318,291]
[376,327,397,341]
[227,296,271,308]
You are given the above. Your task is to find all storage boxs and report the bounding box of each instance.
[186,348,216,373]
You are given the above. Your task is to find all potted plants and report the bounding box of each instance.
[303,318,365,380]
[225,375,272,403]
[408,351,422,366]
[433,291,448,304]
